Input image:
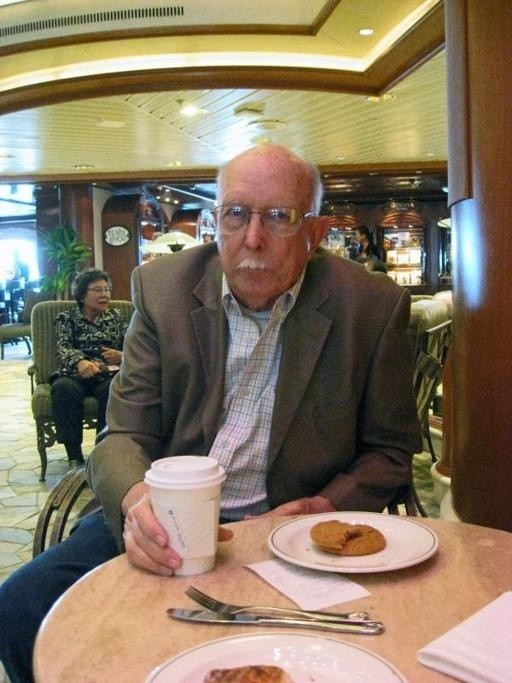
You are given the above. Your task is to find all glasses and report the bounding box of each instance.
[210,204,315,237]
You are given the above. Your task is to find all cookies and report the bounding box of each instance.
[331,524,387,556]
[201,662,286,683]
[309,519,359,554]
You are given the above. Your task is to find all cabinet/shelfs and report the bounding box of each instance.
[101,194,216,301]
[321,198,431,294]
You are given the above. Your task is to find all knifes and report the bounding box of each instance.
[168,607,385,634]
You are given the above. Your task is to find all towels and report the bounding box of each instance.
[416,591,512,683]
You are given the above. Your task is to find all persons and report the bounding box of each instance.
[354,225,383,261]
[0,138,422,681]
[53,265,139,465]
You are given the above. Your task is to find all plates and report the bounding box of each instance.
[145,633,408,683]
[265,510,440,573]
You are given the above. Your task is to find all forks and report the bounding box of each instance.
[183,583,369,622]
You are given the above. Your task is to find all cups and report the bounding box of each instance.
[146,454,228,576]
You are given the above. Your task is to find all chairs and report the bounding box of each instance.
[1,290,136,482]
[409,290,454,517]
[32,467,419,561]
